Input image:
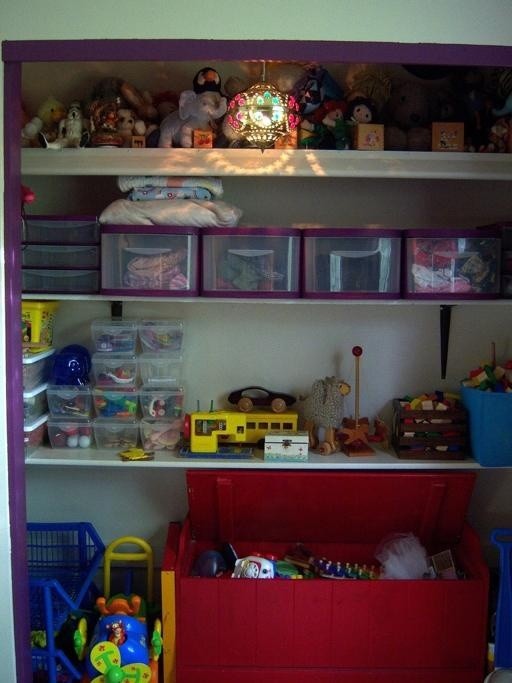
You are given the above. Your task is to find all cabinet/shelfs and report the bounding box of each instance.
[0,35,512,682]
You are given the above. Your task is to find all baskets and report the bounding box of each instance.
[26,521,106,682]
[390,397,467,460]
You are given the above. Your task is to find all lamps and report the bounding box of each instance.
[225,60,303,156]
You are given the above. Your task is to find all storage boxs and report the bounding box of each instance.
[161,464,490,681]
[17,302,188,457]
[460,376,512,467]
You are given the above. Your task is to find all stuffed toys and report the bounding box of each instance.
[22,58,512,153]
